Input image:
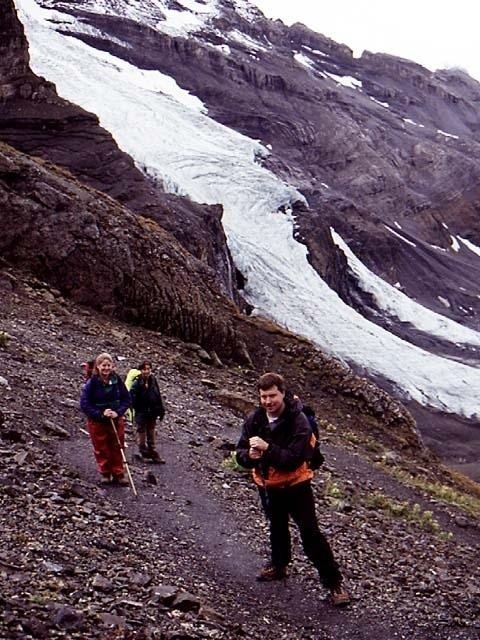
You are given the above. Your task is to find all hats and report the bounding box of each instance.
[137,360,152,370]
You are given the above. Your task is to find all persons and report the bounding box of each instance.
[128,360,165,464]
[79,352,130,487]
[234,371,351,607]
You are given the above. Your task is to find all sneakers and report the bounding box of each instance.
[255,566,286,582]
[329,582,350,606]
[101,474,129,486]
[134,448,166,464]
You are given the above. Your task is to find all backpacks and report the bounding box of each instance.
[124,369,155,422]
[81,361,99,383]
[299,404,325,472]
